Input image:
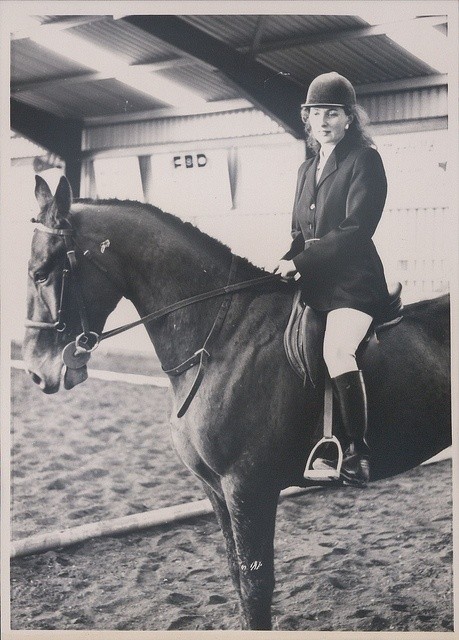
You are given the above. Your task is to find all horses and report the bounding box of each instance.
[25,172,453,632]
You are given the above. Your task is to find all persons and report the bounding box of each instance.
[275,71,388,486]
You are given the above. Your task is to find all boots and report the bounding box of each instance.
[312,370,370,488]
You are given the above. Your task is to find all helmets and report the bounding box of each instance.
[300,71,356,107]
